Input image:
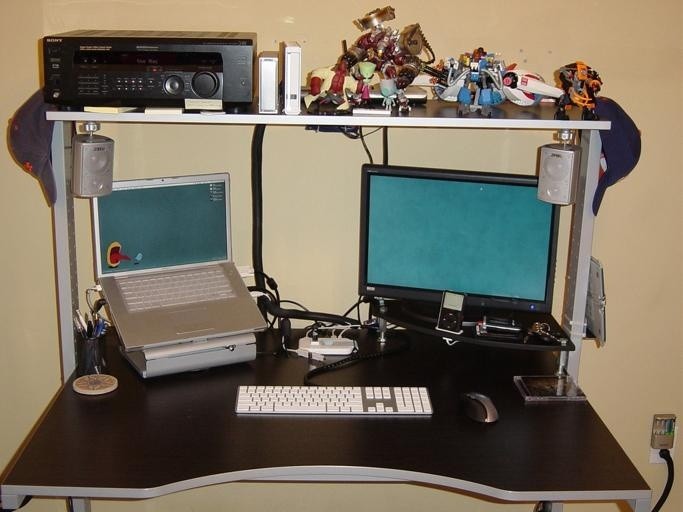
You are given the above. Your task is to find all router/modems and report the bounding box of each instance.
[368,82,427,105]
[283,41,301,115]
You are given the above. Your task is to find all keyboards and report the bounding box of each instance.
[234,385,434,415]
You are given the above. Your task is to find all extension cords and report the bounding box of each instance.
[282,336,354,356]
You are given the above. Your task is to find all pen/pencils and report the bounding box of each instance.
[73,309,108,376]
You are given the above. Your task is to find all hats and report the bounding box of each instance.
[589,95,642,216]
[7,86,58,208]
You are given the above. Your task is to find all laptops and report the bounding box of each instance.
[91,172,267,353]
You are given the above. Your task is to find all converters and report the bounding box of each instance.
[285,329,299,349]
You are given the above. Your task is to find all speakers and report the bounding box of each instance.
[71,134,114,197]
[537,144,582,206]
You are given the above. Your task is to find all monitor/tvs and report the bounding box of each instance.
[358,164,560,328]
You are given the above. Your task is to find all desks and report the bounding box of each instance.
[1,328,654,510]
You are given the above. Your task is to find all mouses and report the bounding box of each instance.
[460,392,498,423]
